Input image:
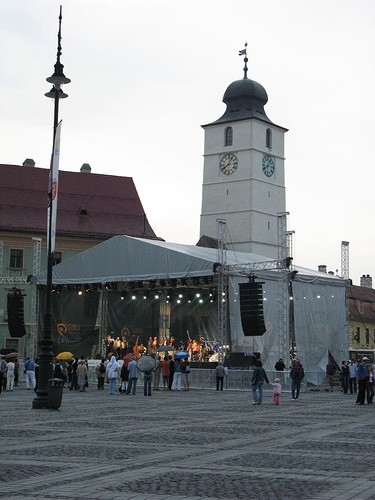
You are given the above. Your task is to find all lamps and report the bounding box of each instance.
[52,277,226,306]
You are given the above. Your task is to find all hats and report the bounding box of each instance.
[363,357,369,360]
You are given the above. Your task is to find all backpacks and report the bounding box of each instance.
[99,363,106,373]
[185,365,191,372]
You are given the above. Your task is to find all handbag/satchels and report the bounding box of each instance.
[24,370,26,374]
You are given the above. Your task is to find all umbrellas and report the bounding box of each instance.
[55,351,74,360]
[5,352,21,358]
[174,352,190,359]
[156,345,176,352]
[136,355,156,372]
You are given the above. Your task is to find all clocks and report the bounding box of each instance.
[262,155,275,178]
[219,153,238,175]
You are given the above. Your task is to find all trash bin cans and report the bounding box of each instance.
[48,378,64,409]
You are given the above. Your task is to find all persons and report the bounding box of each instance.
[214,361,226,391]
[326,360,336,392]
[275,358,286,371]
[290,360,305,401]
[250,359,268,405]
[0,334,218,396]
[339,356,375,406]
[269,378,281,406]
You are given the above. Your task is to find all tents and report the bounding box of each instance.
[35,234,347,392]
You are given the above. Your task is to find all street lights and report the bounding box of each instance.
[32,5,71,409]
